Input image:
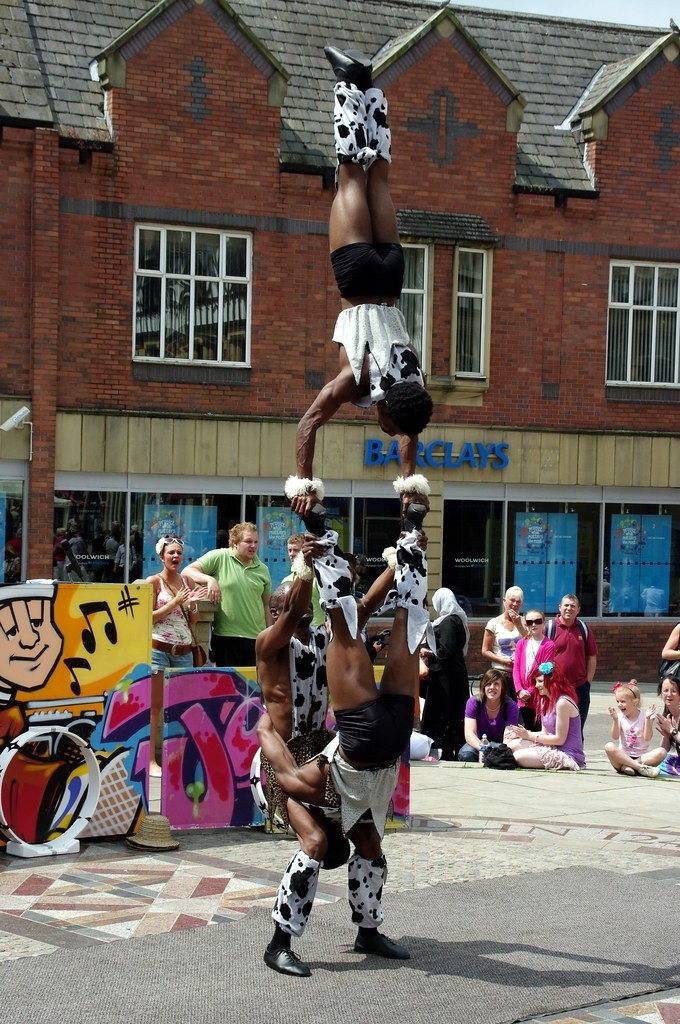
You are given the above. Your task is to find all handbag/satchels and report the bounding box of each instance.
[658,656,680,680]
[482,744,516,770]
[192,645,206,667]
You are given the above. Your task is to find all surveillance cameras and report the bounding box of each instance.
[0,406,30,432]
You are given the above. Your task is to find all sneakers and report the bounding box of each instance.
[621,765,635,776]
[638,764,659,778]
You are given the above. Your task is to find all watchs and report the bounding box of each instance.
[189,602,198,614]
[671,729,679,737]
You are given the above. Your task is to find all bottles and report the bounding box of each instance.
[479,735,490,767]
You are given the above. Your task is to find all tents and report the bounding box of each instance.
[0,480,70,529]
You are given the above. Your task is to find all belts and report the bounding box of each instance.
[152,639,192,656]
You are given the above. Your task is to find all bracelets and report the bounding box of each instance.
[535,733,539,743]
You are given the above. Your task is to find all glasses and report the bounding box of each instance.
[164,538,184,545]
[526,619,544,626]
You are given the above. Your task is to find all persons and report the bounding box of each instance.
[257,501,429,871]
[602,567,669,617]
[283,45,433,519]
[503,660,586,771]
[256,531,429,981]
[512,609,555,731]
[4,506,143,584]
[144,536,207,671]
[604,678,667,778]
[481,586,530,703]
[458,668,519,762]
[654,674,680,776]
[180,521,275,667]
[659,621,680,720]
[541,593,598,746]
[268,532,473,760]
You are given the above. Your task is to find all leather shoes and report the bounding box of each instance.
[324,46,361,80]
[354,934,410,958]
[344,48,372,76]
[263,944,311,976]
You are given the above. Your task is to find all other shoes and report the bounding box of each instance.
[284,489,327,524]
[400,491,428,524]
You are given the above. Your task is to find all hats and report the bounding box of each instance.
[126,815,180,851]
[2,545,16,554]
